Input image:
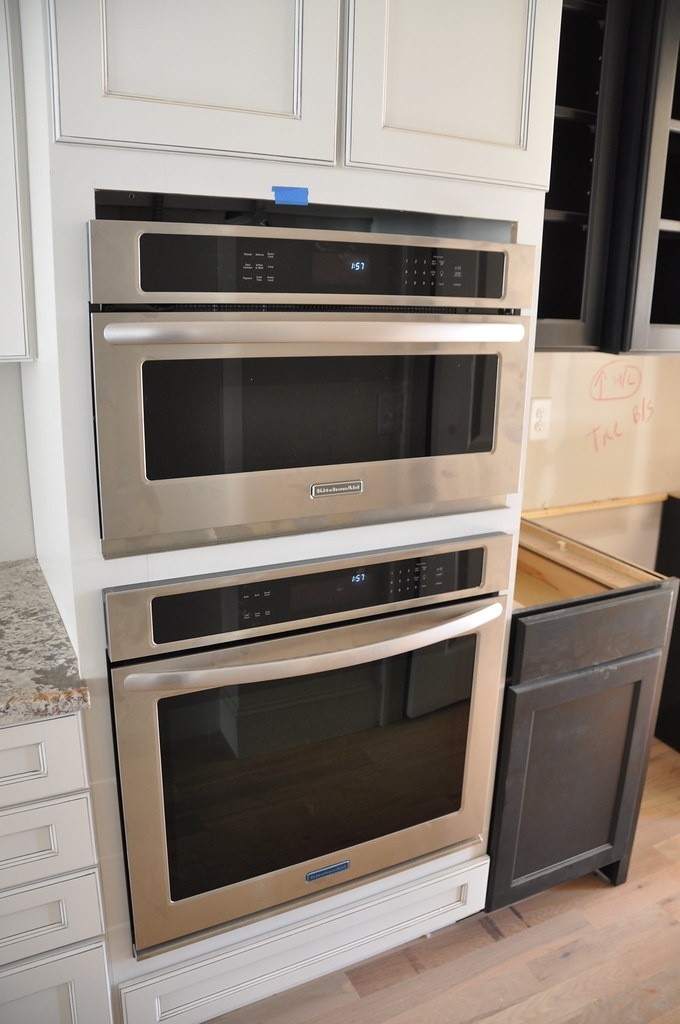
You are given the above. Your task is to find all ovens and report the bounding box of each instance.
[104,533,514,962]
[86,190,538,559]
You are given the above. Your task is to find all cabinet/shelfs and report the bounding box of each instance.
[0,709,115,1024]
[111,855,491,1024]
[17,1,561,190]
[484,518,680,913]
